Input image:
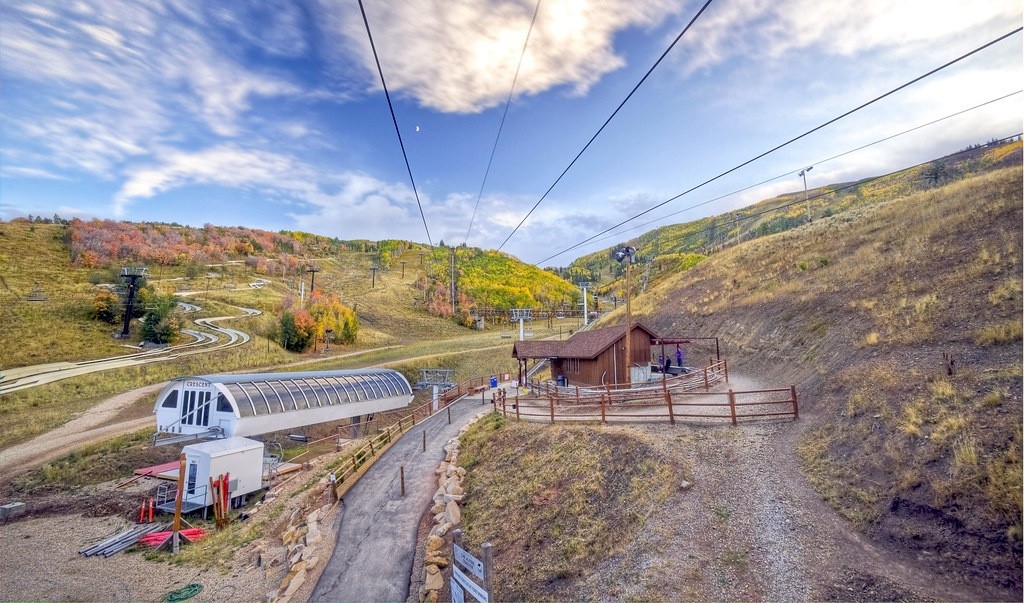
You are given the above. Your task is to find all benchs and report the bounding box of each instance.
[475,384,490,393]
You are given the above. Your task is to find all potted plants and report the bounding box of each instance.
[466,386,474,395]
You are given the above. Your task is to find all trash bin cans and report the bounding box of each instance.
[490,377,497,388]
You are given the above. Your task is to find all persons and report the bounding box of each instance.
[659,356,671,373]
[675,347,684,367]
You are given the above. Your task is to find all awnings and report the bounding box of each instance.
[651,339,694,345]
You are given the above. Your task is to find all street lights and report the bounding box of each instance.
[798,164,813,223]
